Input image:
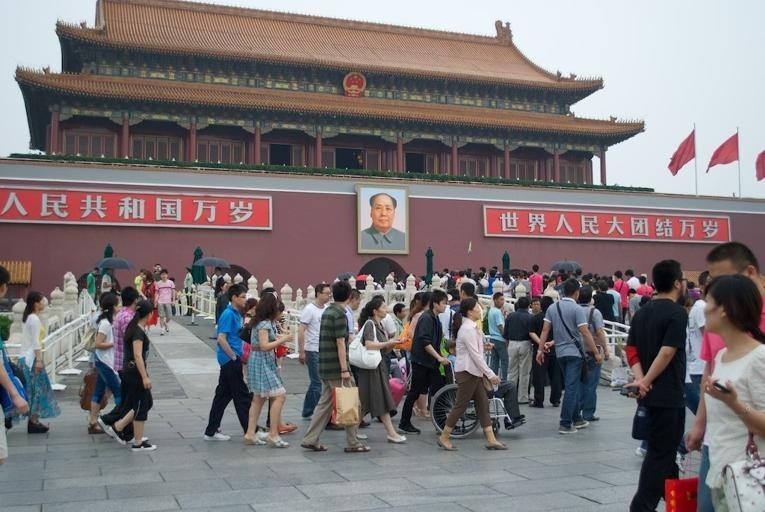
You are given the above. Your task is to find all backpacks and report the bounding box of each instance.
[143,280,154,301]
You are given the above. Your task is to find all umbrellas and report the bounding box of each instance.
[95,257,134,290]
[99,242,115,277]
[425,247,434,274]
[191,246,207,285]
[502,250,510,273]
[549,258,582,274]
[192,254,230,286]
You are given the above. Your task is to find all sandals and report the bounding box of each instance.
[344,445,371,452]
[301,442,329,451]
[88,422,106,434]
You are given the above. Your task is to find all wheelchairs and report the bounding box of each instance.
[430,359,526,439]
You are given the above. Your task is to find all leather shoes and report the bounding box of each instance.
[485,443,509,450]
[437,438,457,451]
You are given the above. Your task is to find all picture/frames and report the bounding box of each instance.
[356,182,409,255]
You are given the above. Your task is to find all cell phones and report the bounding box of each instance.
[713,380,732,393]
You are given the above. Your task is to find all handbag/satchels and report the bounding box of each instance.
[2,375,29,412]
[482,308,491,335]
[394,321,412,351]
[349,337,382,370]
[587,321,596,336]
[331,379,359,427]
[483,375,500,392]
[580,359,589,385]
[240,322,251,344]
[665,476,700,512]
[80,328,98,352]
[78,374,108,410]
[721,458,765,512]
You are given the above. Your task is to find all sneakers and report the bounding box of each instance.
[587,416,599,421]
[573,421,590,428]
[97,415,114,438]
[108,423,126,444]
[398,424,420,434]
[131,442,157,452]
[635,448,648,461]
[528,400,536,407]
[160,331,165,335]
[128,436,148,444]
[675,451,686,473]
[387,434,407,443]
[278,424,297,434]
[204,432,232,441]
[356,434,368,440]
[553,402,559,407]
[558,424,578,434]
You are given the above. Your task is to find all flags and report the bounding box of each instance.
[705,133,739,173]
[667,128,695,177]
[755,150,765,181]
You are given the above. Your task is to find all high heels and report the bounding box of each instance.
[5,417,12,429]
[28,420,49,433]
[266,436,290,448]
[242,436,268,446]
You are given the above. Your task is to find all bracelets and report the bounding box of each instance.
[738,403,752,419]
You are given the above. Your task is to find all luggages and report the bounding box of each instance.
[389,370,412,409]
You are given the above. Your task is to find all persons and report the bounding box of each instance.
[682,270,715,412]
[0,266,160,462]
[683,242,765,512]
[361,193,405,250]
[625,259,689,512]
[203,265,654,453]
[135,264,227,339]
[696,274,765,512]
